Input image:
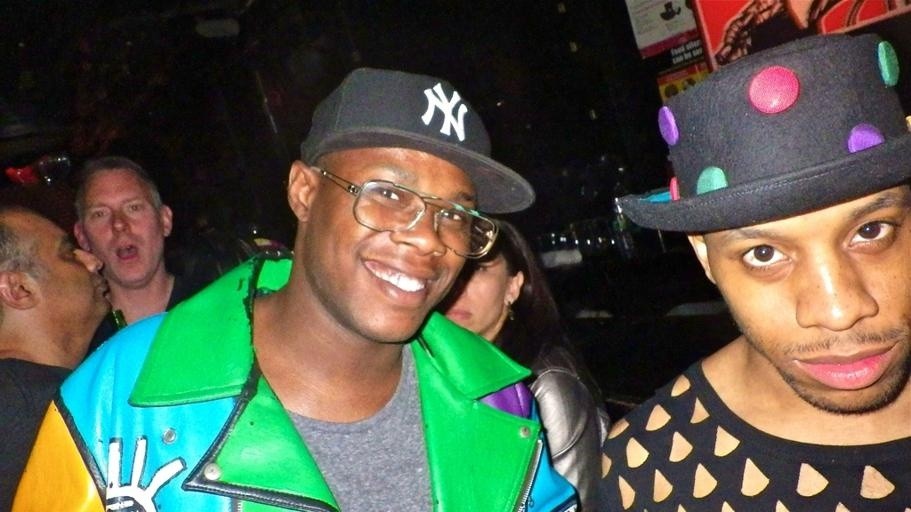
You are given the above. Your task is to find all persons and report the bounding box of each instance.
[440,211,601,512]
[1,202,123,512]
[720,0,911,65]
[68,153,217,339]
[8,64,582,512]
[588,32,911,510]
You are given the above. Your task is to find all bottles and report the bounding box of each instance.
[610,196,637,258]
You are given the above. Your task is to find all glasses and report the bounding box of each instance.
[313,165,499,260]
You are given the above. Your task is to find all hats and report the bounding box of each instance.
[300,67,536,214]
[618,33,910,232]
